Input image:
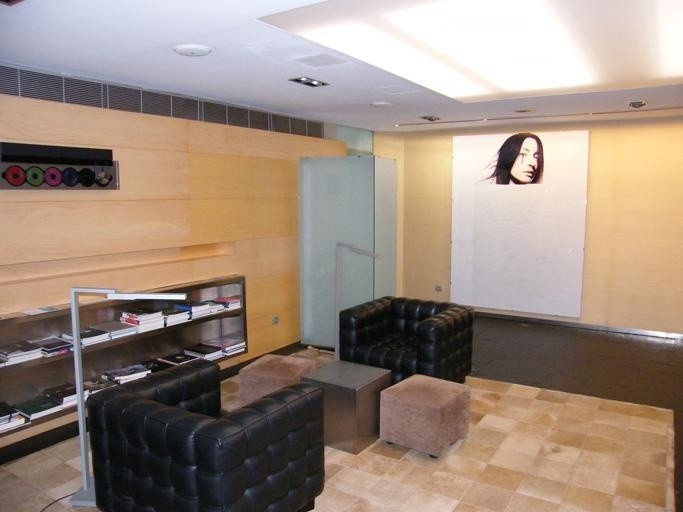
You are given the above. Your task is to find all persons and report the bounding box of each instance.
[472,131,546,187]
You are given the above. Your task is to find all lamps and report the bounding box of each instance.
[69,287,187,508]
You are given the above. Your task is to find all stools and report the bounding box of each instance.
[379,373,471,459]
[239,354,316,408]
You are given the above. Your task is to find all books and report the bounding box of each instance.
[0,294,246,436]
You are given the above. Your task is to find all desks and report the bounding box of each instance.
[301,360,393,455]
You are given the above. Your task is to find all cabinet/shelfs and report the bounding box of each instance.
[0,274,247,448]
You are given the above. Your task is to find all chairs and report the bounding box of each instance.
[86,359,324,512]
[340,296,474,385]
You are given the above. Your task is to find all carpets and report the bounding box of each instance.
[0,345,674,512]
